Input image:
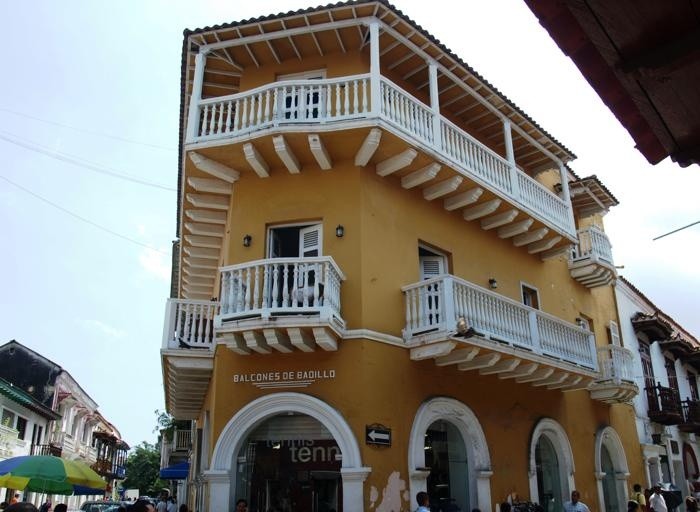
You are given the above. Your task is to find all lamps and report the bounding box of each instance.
[489,278,497,288]
[336,225,344,237]
[243,234,251,247]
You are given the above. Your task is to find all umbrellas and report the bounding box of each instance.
[0,455,108,506]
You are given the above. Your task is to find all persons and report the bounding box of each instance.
[414,484,700,512]
[0,491,189,512]
[236,499,249,512]
